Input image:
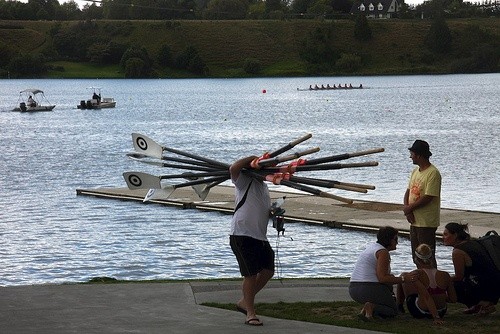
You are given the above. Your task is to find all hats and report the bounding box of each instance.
[408,140,432,156]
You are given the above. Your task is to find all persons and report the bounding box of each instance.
[310,84,362,90]
[92,92,100,103]
[27,95,36,106]
[349,223,500,330]
[229,156,284,326]
[404,140,441,269]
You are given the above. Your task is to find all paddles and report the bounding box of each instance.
[122,132,386,206]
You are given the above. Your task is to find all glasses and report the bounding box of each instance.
[392,237,398,241]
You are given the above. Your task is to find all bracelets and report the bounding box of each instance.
[401,276,405,282]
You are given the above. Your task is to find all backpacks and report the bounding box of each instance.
[470,231,500,271]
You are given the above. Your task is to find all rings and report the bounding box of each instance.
[411,278,413,280]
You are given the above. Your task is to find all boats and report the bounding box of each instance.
[76,86,117,110]
[11,89,56,112]
[297,82,370,91]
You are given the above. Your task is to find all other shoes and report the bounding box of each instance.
[476,304,496,318]
[464,305,481,314]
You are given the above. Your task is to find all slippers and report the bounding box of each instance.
[357,314,376,323]
[236,301,247,315]
[245,317,263,326]
